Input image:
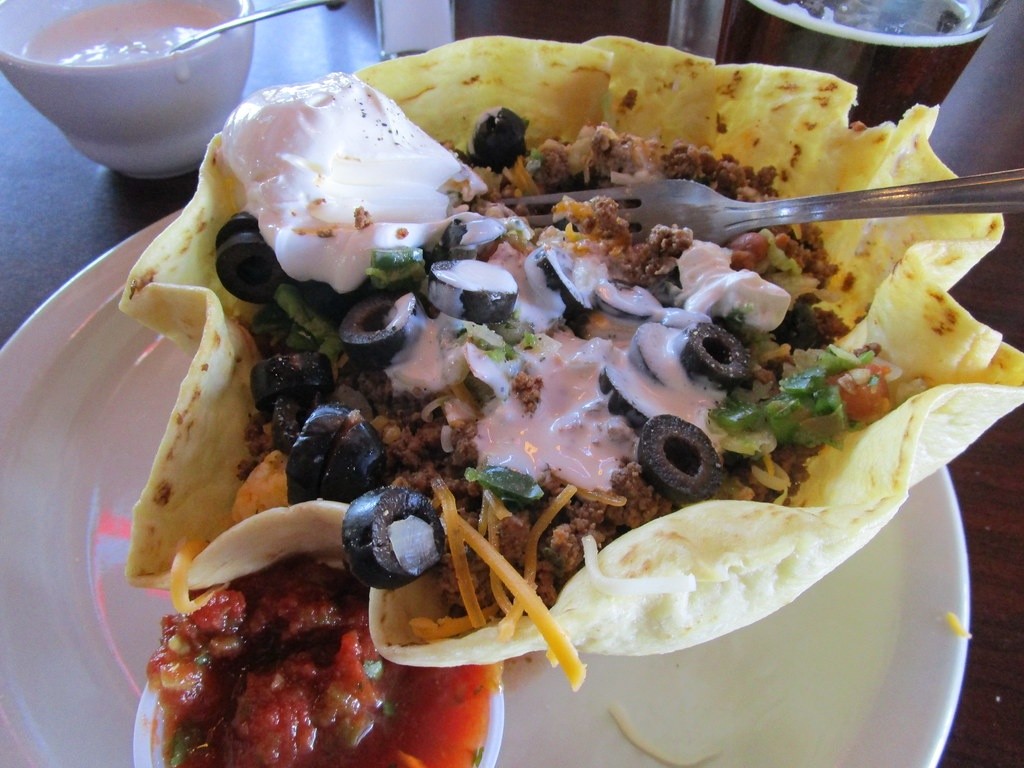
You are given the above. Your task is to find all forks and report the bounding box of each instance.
[499,170,1024,249]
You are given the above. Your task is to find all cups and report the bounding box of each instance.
[373,0,457,62]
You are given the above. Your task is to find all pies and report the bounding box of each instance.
[115,33,1024,666]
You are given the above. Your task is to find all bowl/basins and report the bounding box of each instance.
[1,0,255,180]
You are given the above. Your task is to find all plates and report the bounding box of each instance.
[1,206,970,768]
[748,0,1004,47]
